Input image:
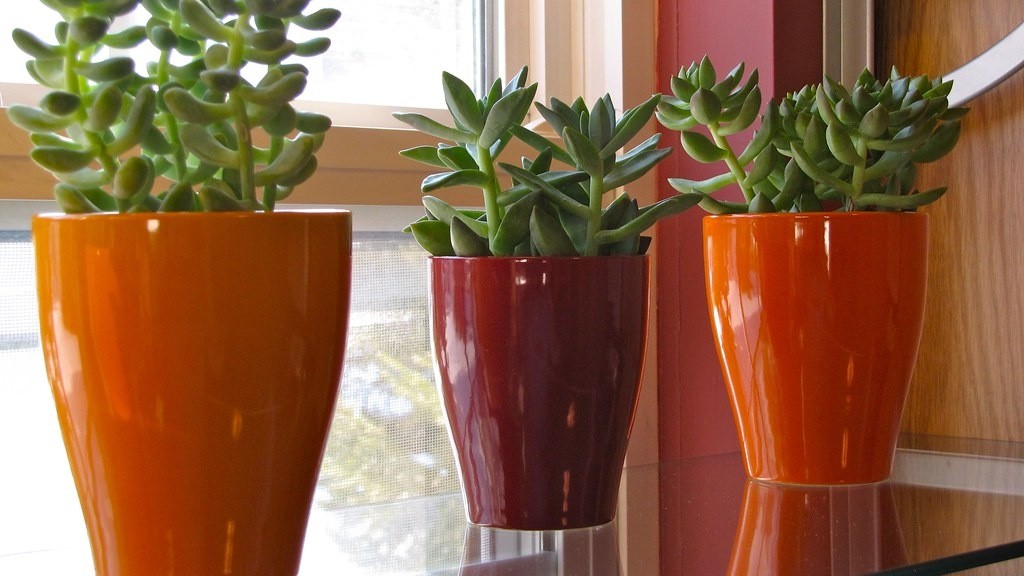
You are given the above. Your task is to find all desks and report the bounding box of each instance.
[311,450,1024,576]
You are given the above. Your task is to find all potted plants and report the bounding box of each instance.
[655,55,972,491]
[5,0,355,576]
[389,65,705,531]
